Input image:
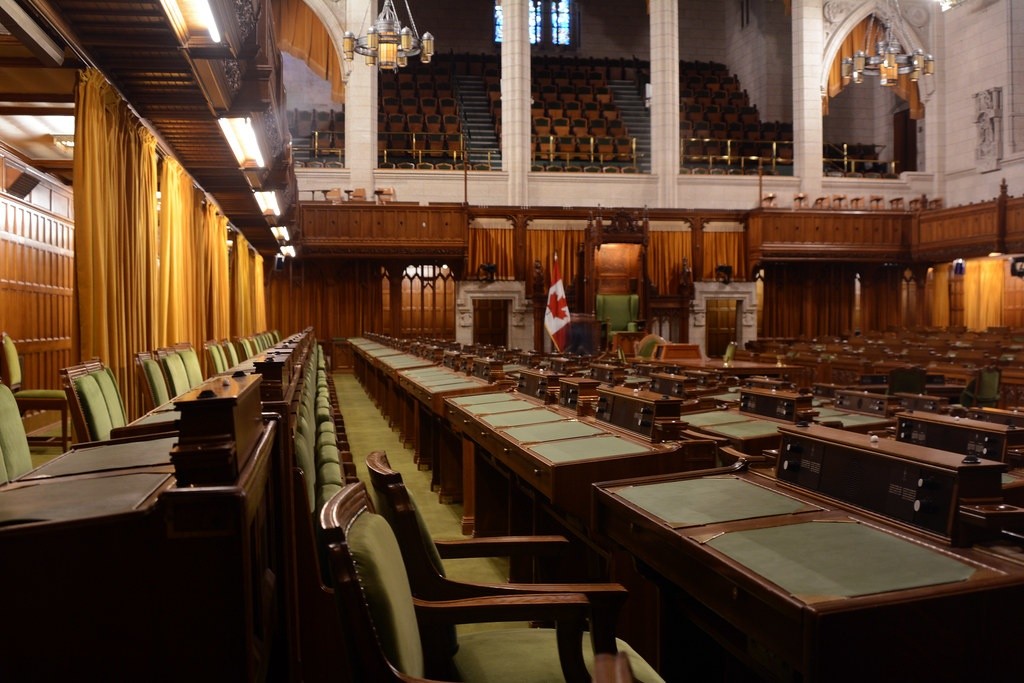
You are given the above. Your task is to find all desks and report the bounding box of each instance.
[347,332,1024,683]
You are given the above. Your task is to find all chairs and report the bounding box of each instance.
[0,329,663,683]
[294,50,791,162]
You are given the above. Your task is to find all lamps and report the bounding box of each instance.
[342,0,434,70]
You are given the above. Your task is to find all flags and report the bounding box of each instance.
[545,258,574,353]
